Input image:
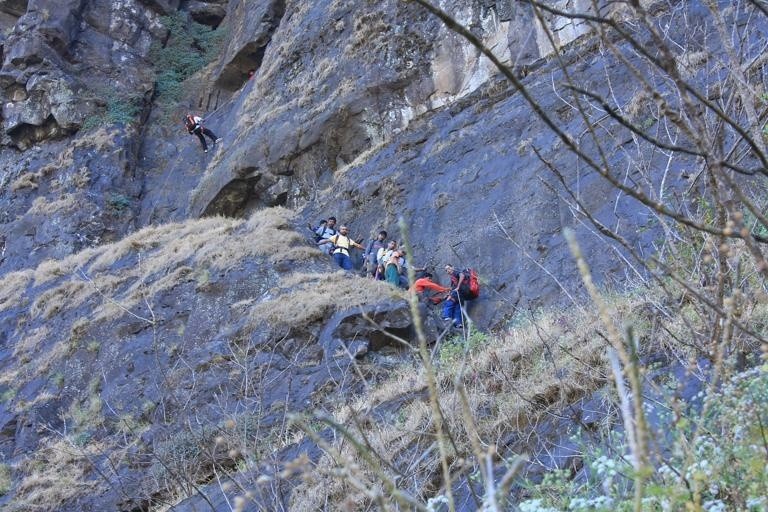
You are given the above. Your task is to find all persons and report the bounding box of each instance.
[248,69,255,79]
[182,111,223,154]
[307,216,471,328]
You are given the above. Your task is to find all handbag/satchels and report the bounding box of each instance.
[462,268,480,301]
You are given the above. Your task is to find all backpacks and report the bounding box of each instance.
[184,115,197,131]
